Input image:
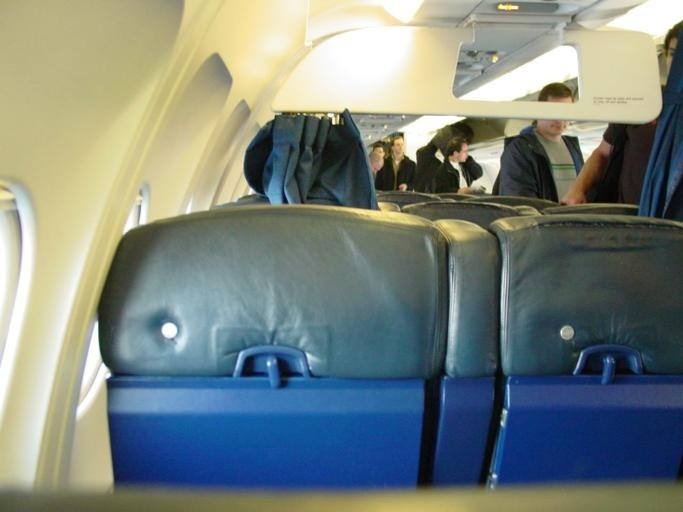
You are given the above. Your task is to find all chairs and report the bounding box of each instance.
[97,204,499,489]
[487,214,681,484]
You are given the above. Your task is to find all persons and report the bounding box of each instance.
[496,81,585,205]
[560,19,682,204]
[365,121,485,196]
